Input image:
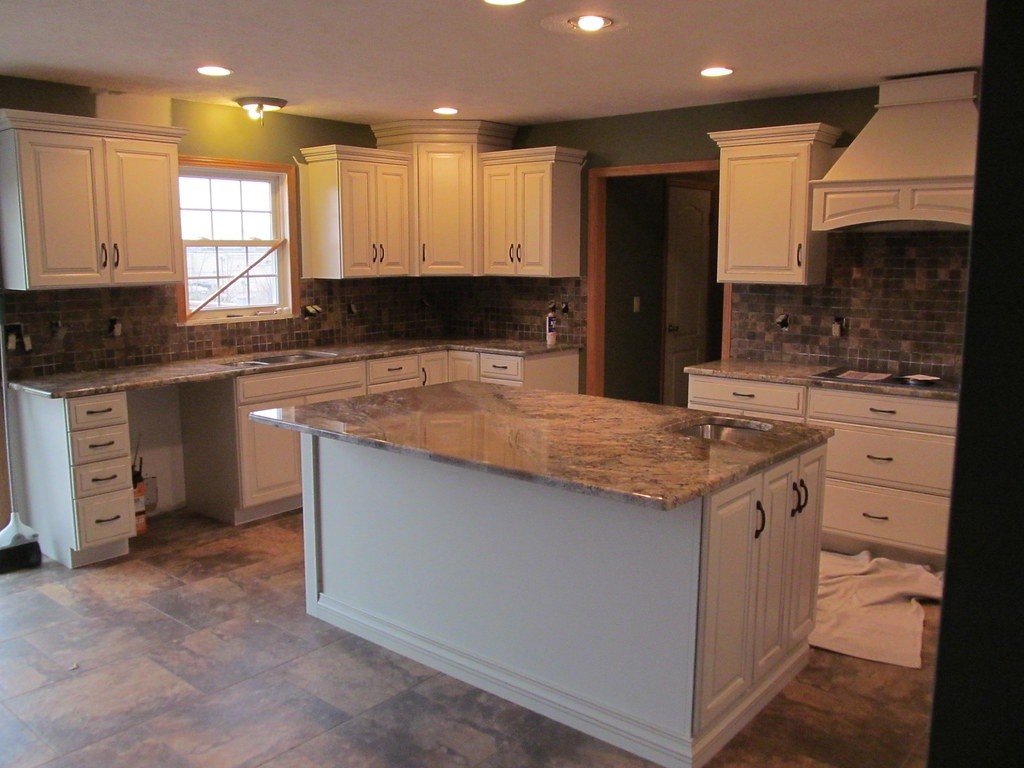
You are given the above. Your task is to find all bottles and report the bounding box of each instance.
[546,308,556,345]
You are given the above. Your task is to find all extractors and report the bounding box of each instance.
[810,71,980,232]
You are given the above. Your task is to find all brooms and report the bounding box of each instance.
[0,336,43,575]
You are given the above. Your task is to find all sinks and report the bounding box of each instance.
[664,416,773,447]
[218,361,268,370]
[255,351,337,364]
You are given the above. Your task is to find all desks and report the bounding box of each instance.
[249,378,835,768]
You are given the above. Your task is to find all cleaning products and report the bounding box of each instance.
[543,310,559,345]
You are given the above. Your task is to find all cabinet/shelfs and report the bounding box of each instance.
[0,108,191,292]
[479,145,590,279]
[291,144,414,278]
[370,121,520,277]
[445,349,584,396]
[8,390,137,571]
[178,351,449,526]
[705,120,844,287]
[684,375,961,572]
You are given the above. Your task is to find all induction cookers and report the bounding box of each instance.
[810,366,960,393]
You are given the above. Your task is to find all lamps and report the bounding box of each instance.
[236,98,288,126]
